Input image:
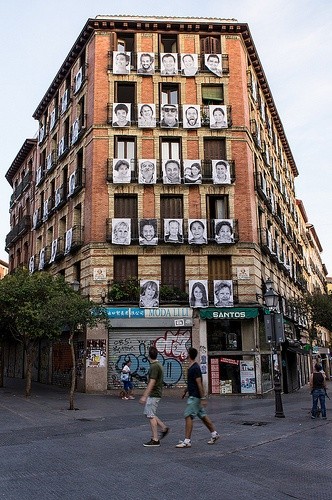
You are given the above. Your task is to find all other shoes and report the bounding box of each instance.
[130,396,135,399]
[159,428,170,440]
[176,439,192,448]
[311,415,316,419]
[143,438,161,447]
[207,431,221,444]
[122,397,129,400]
[322,416,326,419]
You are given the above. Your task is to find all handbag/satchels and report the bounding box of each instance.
[121,372,130,381]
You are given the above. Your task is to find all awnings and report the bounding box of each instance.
[198,307,259,319]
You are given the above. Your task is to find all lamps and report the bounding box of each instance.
[71,281,89,298]
[256,278,279,308]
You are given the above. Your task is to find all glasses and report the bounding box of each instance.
[162,108,176,113]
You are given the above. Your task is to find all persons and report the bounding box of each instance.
[215,221,234,243]
[164,220,183,241]
[139,281,159,307]
[189,221,207,243]
[308,363,329,420]
[161,104,227,128]
[113,221,130,244]
[164,160,230,185]
[161,53,222,76]
[114,104,156,127]
[116,53,154,73]
[190,282,208,307]
[140,218,158,244]
[175,348,220,448]
[113,160,156,183]
[215,280,233,306]
[138,346,170,446]
[120,361,135,400]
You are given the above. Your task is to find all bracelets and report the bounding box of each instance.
[201,397,206,400]
